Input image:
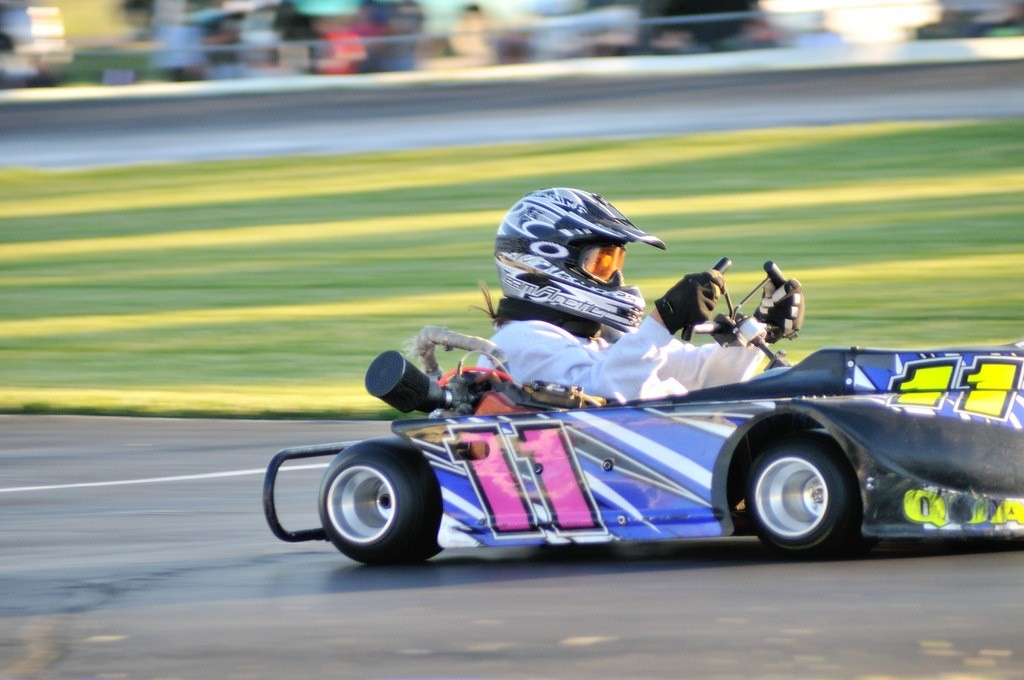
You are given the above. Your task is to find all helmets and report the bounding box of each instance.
[493,187,668,339]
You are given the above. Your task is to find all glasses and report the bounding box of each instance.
[578,243,627,286]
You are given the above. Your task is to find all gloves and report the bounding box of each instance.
[653,270,727,338]
[750,278,804,343]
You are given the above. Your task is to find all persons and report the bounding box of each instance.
[477,185,804,402]
[154,1,839,79]
